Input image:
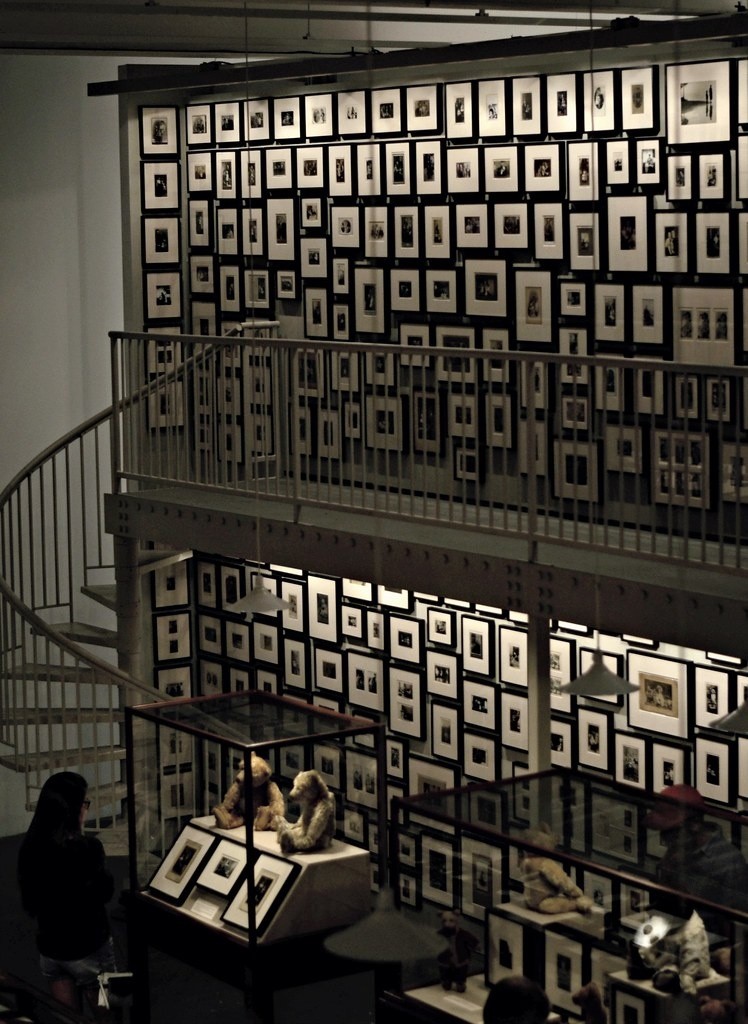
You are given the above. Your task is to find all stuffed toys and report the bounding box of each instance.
[515,817,596,918]
[572,932,748,1024]
[270,767,338,856]
[211,754,286,832]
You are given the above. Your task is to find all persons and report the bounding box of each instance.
[665,230,678,256]
[483,977,551,1024]
[645,785,748,977]
[466,218,479,233]
[15,770,118,1023]
[428,907,481,994]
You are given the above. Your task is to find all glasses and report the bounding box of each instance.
[82,799,91,808]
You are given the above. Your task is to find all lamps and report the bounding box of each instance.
[221,518,295,613]
[708,670,748,734]
[554,571,640,696]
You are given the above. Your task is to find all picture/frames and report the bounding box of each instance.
[484,906,542,991]
[542,922,598,1022]
[609,983,662,1024]
[140,57,748,938]
